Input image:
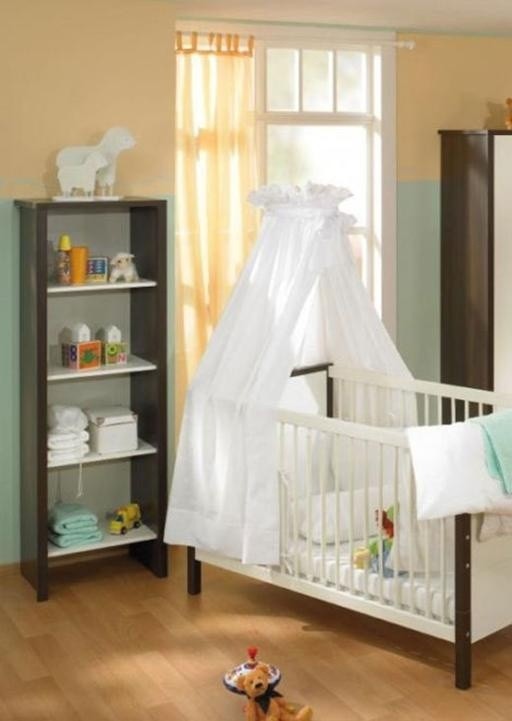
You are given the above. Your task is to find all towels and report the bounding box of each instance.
[47,429,89,450]
[47,444,90,463]
[49,501,97,534]
[480,408,512,495]
[48,532,102,548]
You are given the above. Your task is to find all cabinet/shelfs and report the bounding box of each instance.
[15,194,171,602]
[436,129,512,424]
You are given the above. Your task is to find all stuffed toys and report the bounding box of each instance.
[108,252,140,282]
[234,662,312,720]
[350,505,404,576]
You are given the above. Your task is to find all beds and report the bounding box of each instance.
[164,181,512,691]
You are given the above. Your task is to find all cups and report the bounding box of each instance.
[70,247,88,285]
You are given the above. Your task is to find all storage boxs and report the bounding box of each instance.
[88,405,139,453]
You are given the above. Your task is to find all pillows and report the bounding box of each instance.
[290,483,407,545]
